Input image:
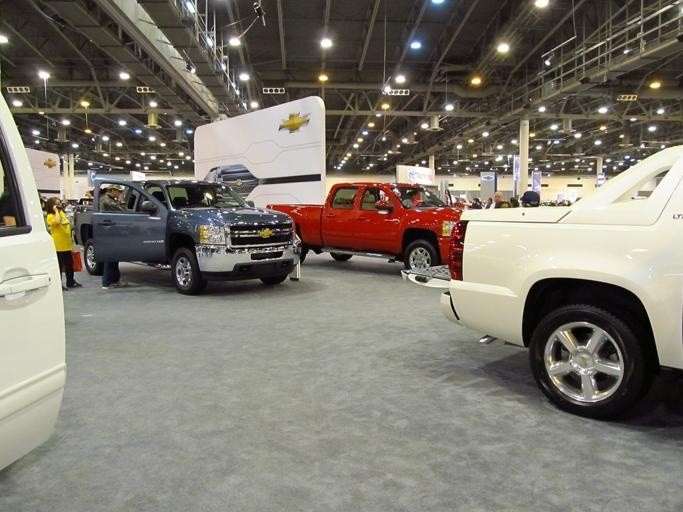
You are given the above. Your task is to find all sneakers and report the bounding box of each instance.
[60,277,82,292]
[100,279,128,291]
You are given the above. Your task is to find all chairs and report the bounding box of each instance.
[333,193,376,210]
[151,190,205,209]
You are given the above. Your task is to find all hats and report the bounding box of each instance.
[102,183,126,192]
[519,191,540,207]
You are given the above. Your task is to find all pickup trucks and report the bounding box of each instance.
[440,142,682,418]
[72,176,303,295]
[266,181,467,271]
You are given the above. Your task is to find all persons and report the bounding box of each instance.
[44,197,82,290]
[99,183,128,290]
[470,191,539,209]
